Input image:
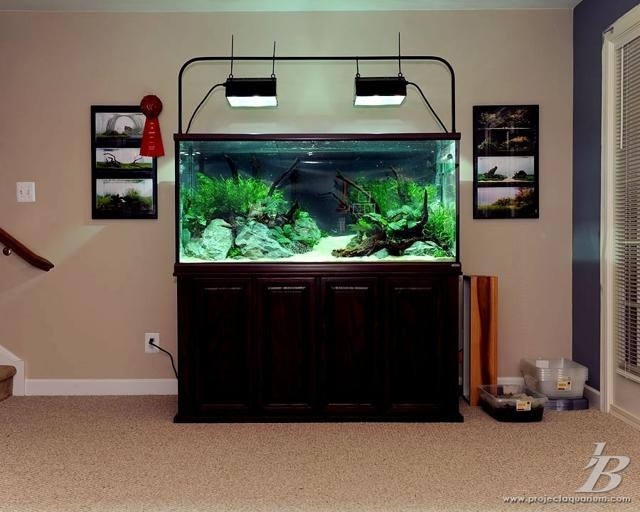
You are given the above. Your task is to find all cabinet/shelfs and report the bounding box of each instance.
[317,262,465,425]
[171,263,317,426]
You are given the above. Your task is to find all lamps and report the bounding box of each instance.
[184,34,280,135]
[349,31,454,135]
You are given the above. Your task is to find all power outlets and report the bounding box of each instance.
[144,332,160,355]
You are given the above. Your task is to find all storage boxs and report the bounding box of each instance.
[476,384,551,425]
[515,354,591,401]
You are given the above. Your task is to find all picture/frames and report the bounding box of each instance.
[88,102,159,221]
[472,102,540,220]
